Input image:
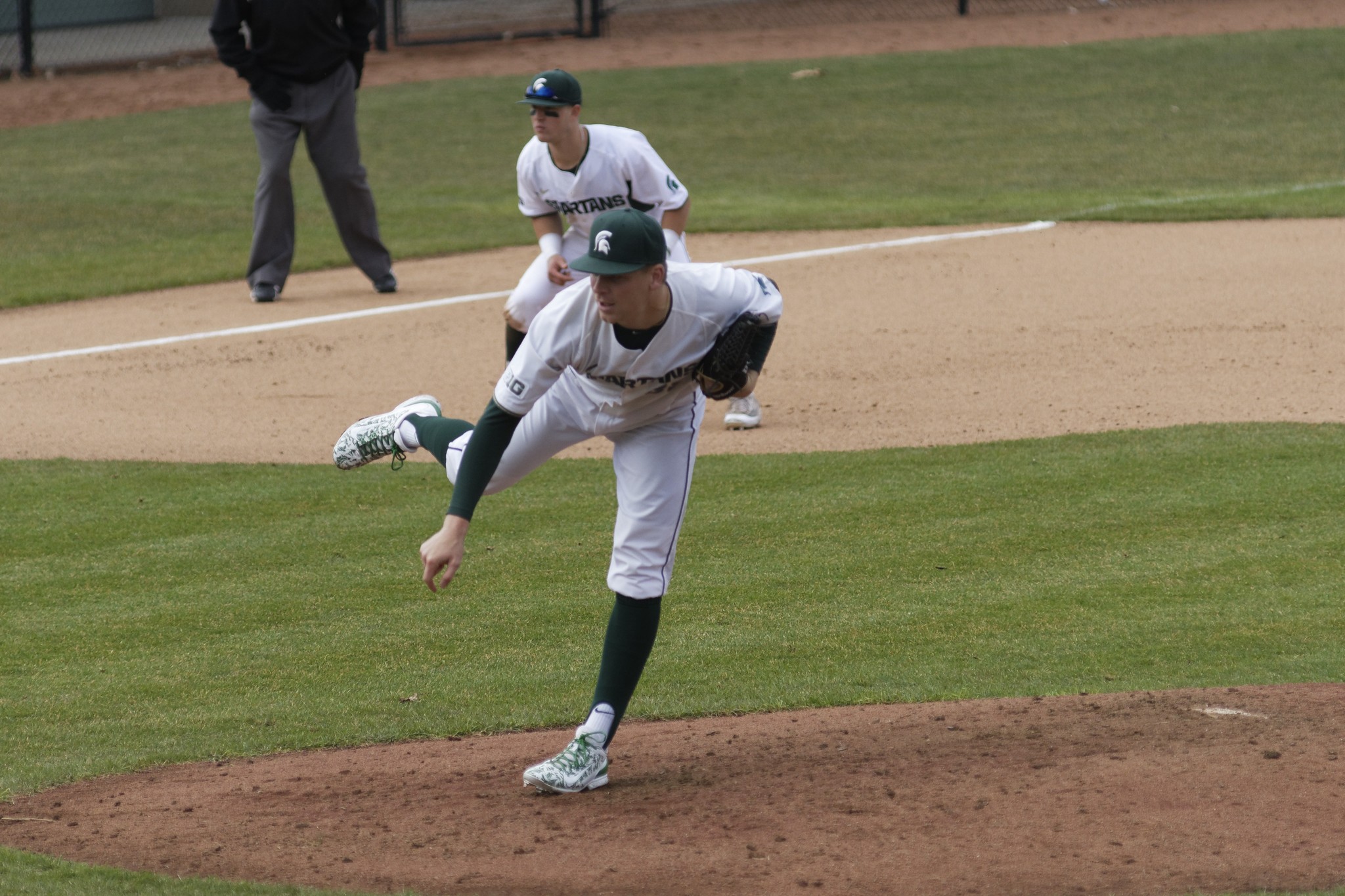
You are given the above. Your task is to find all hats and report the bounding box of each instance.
[569,207,667,276]
[516,69,582,108]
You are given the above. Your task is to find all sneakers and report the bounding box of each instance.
[523,726,609,793]
[724,392,762,431]
[333,395,442,471]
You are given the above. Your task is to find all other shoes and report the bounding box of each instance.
[250,282,278,302]
[370,273,396,293]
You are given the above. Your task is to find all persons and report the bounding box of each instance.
[204,0,396,303]
[330,204,784,794]
[499,67,764,428]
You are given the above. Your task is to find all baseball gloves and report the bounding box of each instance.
[694,312,762,400]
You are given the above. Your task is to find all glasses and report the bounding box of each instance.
[524,85,567,102]
